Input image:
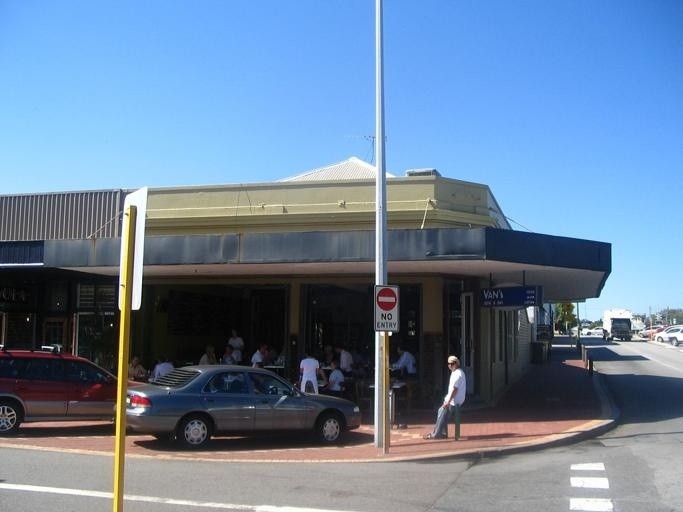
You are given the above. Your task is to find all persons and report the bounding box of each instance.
[151,355,174,380]
[389,343,416,382]
[198,327,370,398]
[423,355,466,439]
[128,355,147,378]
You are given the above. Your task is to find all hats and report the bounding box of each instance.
[448,356,460,366]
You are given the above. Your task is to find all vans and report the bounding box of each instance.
[0,342,147,432]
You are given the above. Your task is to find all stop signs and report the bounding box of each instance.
[373,284,401,332]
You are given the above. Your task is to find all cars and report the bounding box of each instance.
[638,324,682,346]
[111,365,362,450]
[584,326,604,335]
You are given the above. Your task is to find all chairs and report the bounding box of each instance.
[336,373,411,409]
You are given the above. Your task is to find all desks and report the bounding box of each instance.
[321,365,333,377]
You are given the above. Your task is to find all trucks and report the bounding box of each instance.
[602,308,633,341]
